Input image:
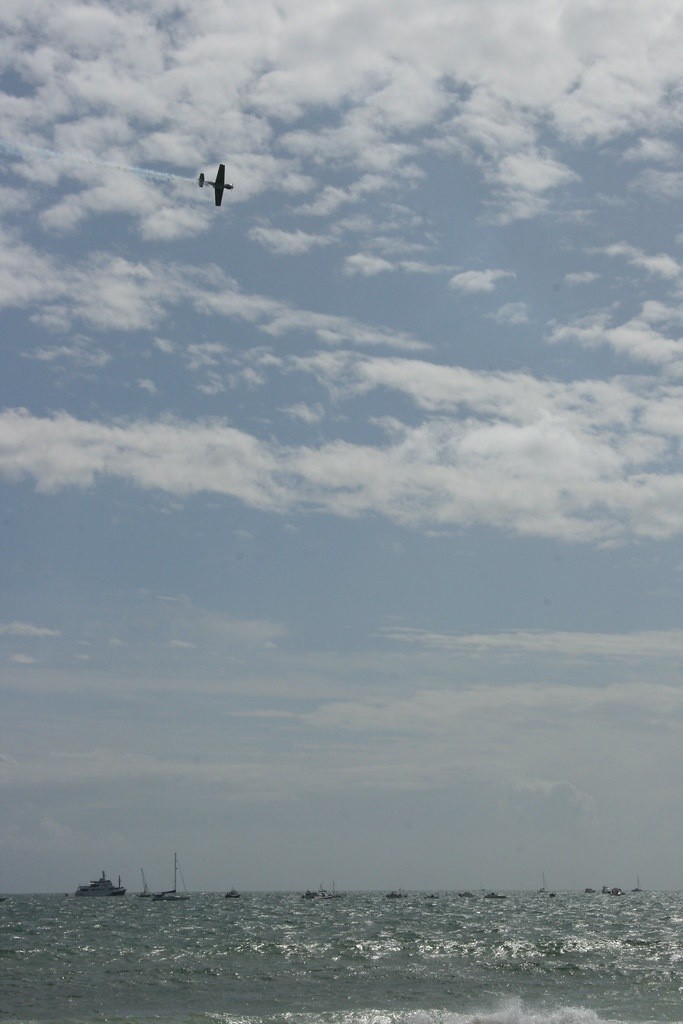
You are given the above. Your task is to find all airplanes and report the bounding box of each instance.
[197,164,233,206]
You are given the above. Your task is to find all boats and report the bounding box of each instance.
[484,891,506,899]
[153,852,191,901]
[137,867,153,898]
[631,874,643,892]
[73,870,127,898]
[298,880,341,901]
[601,886,611,894]
[539,871,549,894]
[225,886,241,899]
[459,892,475,898]
[385,890,408,898]
[423,894,440,900]
[612,887,625,895]
[584,888,596,894]
